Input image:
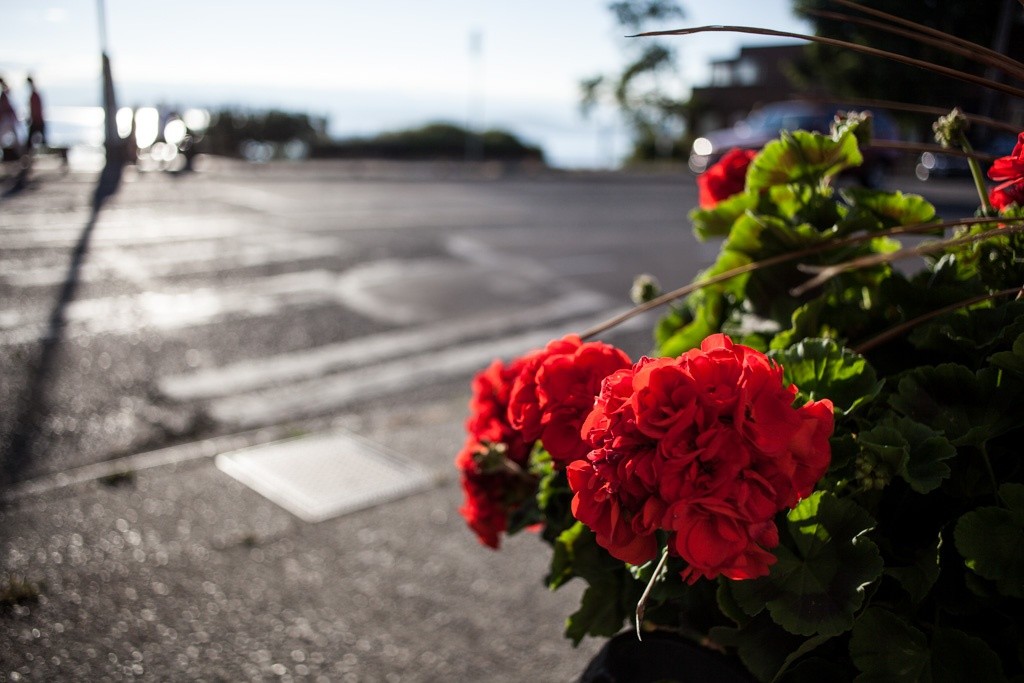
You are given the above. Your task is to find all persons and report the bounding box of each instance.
[0,77,68,164]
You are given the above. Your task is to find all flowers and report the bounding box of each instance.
[459,25,1023,683]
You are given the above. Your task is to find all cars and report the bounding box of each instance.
[688,101,917,189]
